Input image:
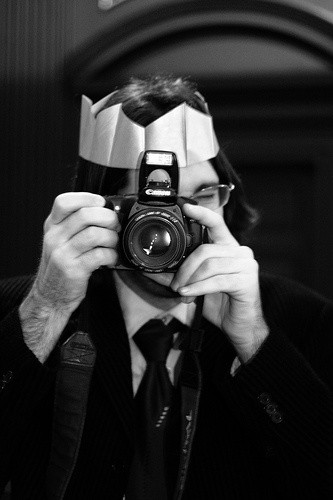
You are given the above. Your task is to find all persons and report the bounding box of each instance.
[0,72,333,500]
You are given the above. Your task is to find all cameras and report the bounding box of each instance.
[101,150,209,273]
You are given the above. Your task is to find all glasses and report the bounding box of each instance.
[105,184,234,222]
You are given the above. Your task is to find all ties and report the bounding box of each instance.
[133,319,178,500]
